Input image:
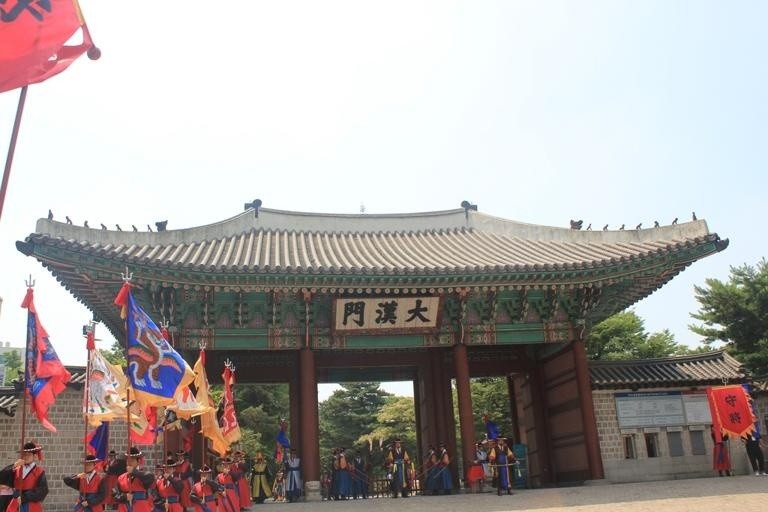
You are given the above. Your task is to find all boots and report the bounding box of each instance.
[288,497,298,502]
[432,489,452,495]
[333,494,367,500]
[255,499,264,504]
[498,487,514,496]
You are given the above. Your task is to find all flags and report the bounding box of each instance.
[0,0,93,94]
[21,283,243,460]
[706,384,760,443]
[274,430,290,464]
[486,419,497,440]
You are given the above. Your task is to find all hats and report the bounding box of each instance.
[253,452,266,459]
[332,447,345,455]
[108,451,118,456]
[425,441,448,451]
[392,490,409,498]
[199,464,212,473]
[390,439,402,446]
[124,447,143,457]
[163,449,185,467]
[475,435,508,446]
[221,450,247,465]
[17,442,42,453]
[81,454,98,463]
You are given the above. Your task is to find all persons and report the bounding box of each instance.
[331,447,354,500]
[476,442,492,491]
[422,444,443,495]
[741,430,768,476]
[272,470,285,501]
[0,441,50,512]
[250,452,272,504]
[283,448,303,503]
[487,434,520,496]
[710,422,732,476]
[352,451,368,499]
[432,441,454,495]
[385,439,412,498]
[63,451,252,512]
